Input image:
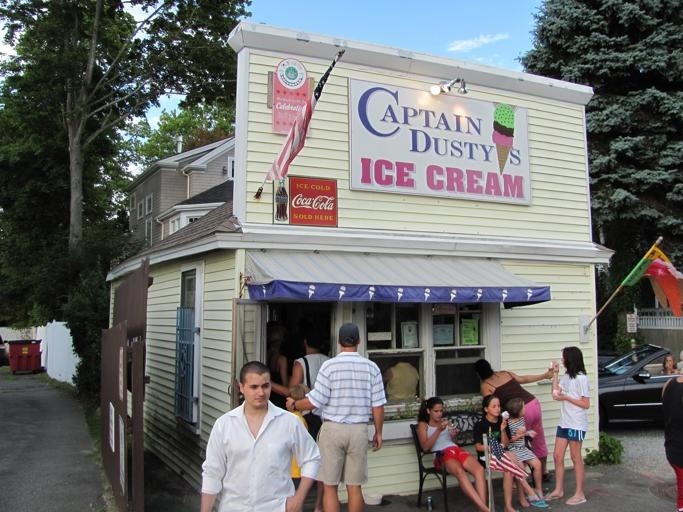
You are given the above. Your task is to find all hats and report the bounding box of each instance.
[339,323,359,342]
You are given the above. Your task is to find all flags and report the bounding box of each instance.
[623,247,683,317]
[264,53,343,184]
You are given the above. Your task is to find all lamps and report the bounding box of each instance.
[430,77,468,96]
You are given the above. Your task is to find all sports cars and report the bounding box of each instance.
[597,343,682,429]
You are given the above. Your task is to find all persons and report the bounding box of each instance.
[266,326,332,489]
[200,361,320,512]
[286,324,386,511]
[545,347,591,504]
[383,356,419,403]
[475,358,554,511]
[662,375,683,512]
[418,396,489,511]
[658,354,682,375]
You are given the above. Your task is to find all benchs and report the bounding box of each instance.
[410,408,534,512]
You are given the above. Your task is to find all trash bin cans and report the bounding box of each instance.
[8,339,43,375]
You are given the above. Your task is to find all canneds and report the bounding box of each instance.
[427,496,433,512]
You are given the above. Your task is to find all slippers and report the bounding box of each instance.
[545,492,560,500]
[565,497,587,505]
[529,492,548,508]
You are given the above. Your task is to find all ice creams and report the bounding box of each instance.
[494,99,516,177]
[500,410,511,426]
